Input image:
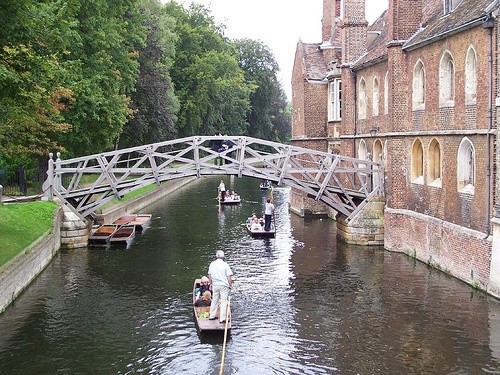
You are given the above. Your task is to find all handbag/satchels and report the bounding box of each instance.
[218,148,226,158]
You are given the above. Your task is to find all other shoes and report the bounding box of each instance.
[220,320,229,323]
[209,317,218,320]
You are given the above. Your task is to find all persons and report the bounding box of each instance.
[249,213,265,230]
[260,179,269,187]
[208,250,233,323]
[209,131,235,170]
[217,180,240,200]
[193,276,212,307]
[264,199,276,231]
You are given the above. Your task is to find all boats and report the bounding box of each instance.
[88,224,123,245]
[109,223,136,245]
[112,214,136,226]
[218,193,242,202]
[193,278,232,334]
[259,181,272,190]
[246,216,277,235]
[122,213,153,232]
